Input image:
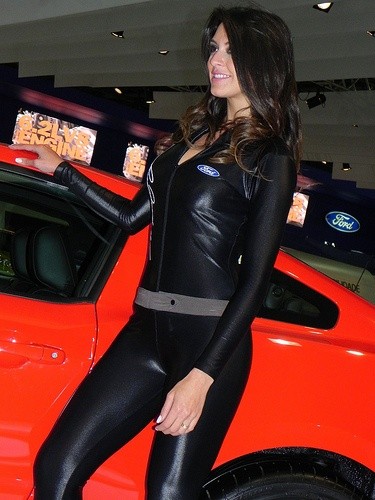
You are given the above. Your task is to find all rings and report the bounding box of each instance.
[181,423,189,428]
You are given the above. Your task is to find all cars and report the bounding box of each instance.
[0,143,375,500]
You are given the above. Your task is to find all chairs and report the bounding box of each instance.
[8,225,77,299]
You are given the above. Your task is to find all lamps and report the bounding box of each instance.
[110,31,126,39]
[306,91,329,108]
[341,162,352,172]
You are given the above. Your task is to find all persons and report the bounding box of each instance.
[8,5,303,500]
